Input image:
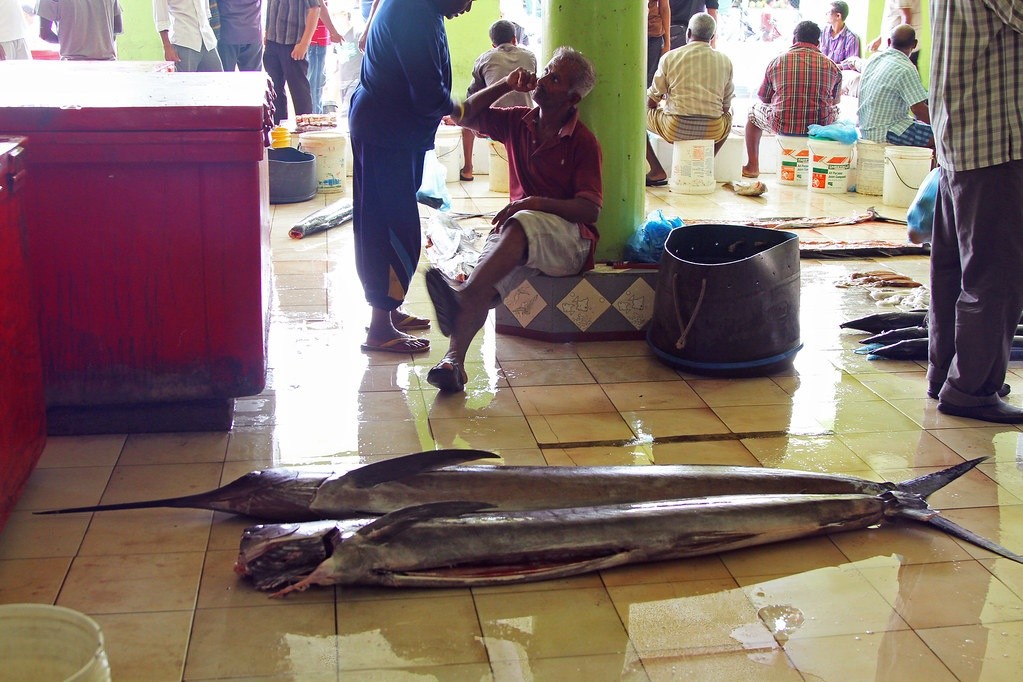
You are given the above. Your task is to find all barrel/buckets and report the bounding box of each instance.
[646,224,803,372]
[0,604,112,682]
[488,141,510,192]
[807,135,854,194]
[857,138,897,196]
[776,133,809,186]
[271,127,290,148]
[290,119,354,193]
[268,147,316,204]
[882,146,934,207]
[31,51,60,61]
[668,140,716,194]
[434,125,465,182]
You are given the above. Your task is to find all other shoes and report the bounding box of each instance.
[927,377,1023,423]
[742,166,758,177]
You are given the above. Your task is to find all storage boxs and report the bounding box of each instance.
[646,129,743,182]
[291,130,355,177]
[435,126,490,175]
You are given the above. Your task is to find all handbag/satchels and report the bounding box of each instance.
[417,150,451,214]
[907,168,940,244]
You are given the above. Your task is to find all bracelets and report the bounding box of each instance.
[456,101,465,123]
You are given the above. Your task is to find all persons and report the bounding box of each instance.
[855,23,935,148]
[209,0,262,72]
[153,0,225,72]
[924,0,1023,424]
[816,0,860,70]
[0,0,34,60]
[647,0,719,89]
[307,0,345,114]
[424,45,603,393]
[646,12,736,186]
[742,21,843,178]
[728,0,800,40]
[263,0,323,126]
[347,0,474,354]
[32,0,125,61]
[866,0,921,71]
[459,19,537,181]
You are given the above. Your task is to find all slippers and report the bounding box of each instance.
[645,174,670,186]
[428,361,470,394]
[426,265,461,338]
[460,168,474,181]
[361,332,430,352]
[364,313,430,331]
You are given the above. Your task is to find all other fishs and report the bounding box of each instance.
[288,197,353,239]
[840,309,1023,335]
[858,325,1023,345]
[868,336,1023,360]
[723,180,767,196]
